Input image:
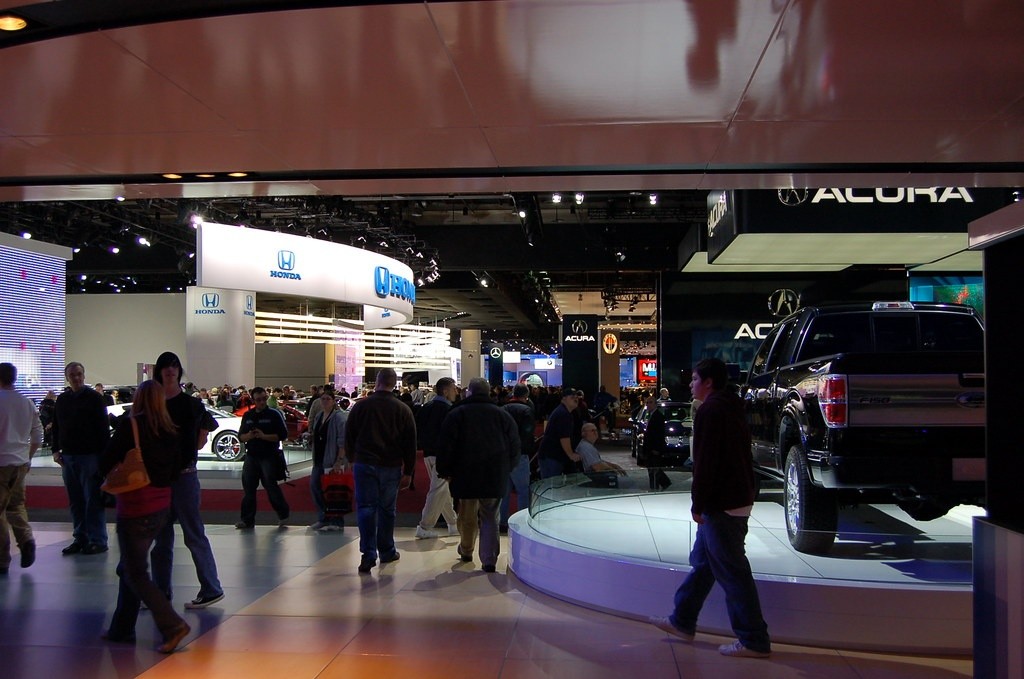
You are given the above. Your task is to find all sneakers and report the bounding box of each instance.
[184,590,226,609]
[648,616,695,641]
[719,639,772,658]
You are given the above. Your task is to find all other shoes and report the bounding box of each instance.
[462,555,472,561]
[311,520,344,532]
[157,622,190,652]
[235,520,253,528]
[101,631,137,645]
[20,540,36,568]
[448,524,461,535]
[358,559,376,572]
[379,552,400,564]
[415,524,439,539]
[482,564,495,573]
[61,542,108,554]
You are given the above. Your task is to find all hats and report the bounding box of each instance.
[559,388,582,397]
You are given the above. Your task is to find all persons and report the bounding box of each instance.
[40,364,672,540]
[434,377,520,571]
[137,351,225,608]
[0,363,42,575]
[346,368,416,571]
[98,380,190,653]
[51,362,112,554]
[649,360,773,658]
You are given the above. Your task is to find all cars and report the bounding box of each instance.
[628,401,691,466]
[234,382,377,450]
[106,399,285,463]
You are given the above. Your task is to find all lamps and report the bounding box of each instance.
[20,187,440,293]
[602,250,640,312]
[478,266,564,355]
[510,188,662,220]
[0,9,30,32]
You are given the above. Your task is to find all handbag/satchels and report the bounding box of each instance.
[100,416,152,495]
[271,448,290,482]
[320,467,355,516]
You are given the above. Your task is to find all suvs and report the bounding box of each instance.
[740,302,985,555]
